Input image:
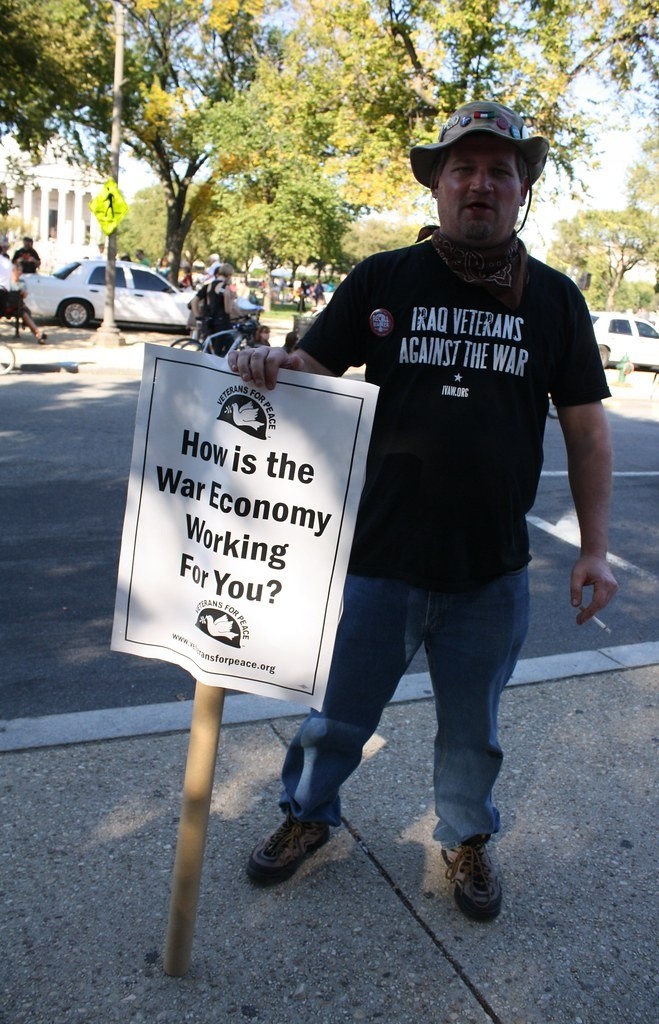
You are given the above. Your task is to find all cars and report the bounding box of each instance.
[17,259,195,330]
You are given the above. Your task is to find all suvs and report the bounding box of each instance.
[589,311,659,369]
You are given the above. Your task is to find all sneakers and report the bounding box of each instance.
[245,805,330,884]
[441,842,502,923]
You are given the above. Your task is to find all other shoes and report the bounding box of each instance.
[35,333,47,346]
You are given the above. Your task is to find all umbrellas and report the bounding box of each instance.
[270,268,292,277]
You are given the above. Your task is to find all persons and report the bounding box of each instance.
[120,252,131,261]
[0,231,48,345]
[156,257,172,278]
[11,237,41,273]
[327,280,335,292]
[227,101,618,922]
[207,254,220,277]
[313,278,323,306]
[180,265,193,288]
[185,263,236,357]
[93,244,105,260]
[136,250,151,266]
[296,276,310,313]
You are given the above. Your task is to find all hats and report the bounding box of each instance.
[205,261,222,276]
[410,101,550,189]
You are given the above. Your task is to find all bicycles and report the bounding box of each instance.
[0,341,15,375]
[170,310,261,360]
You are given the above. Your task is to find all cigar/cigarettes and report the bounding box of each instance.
[579,606,611,634]
